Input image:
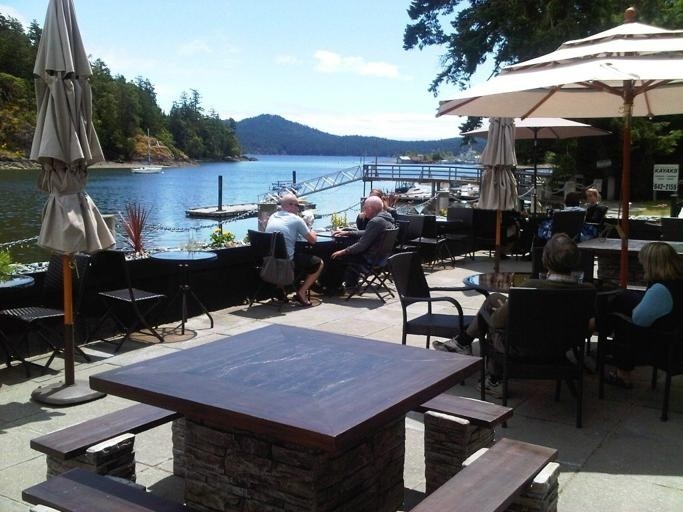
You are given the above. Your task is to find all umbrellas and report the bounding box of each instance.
[24,1,116,388]
[434,8,682,289]
[477,117,520,272]
[460,116,612,214]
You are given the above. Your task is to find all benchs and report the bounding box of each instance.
[26,399,179,487]
[406,391,514,498]
[20,464,207,511]
[405,431,561,511]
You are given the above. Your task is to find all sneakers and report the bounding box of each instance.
[433,335,472,355]
[475,375,503,399]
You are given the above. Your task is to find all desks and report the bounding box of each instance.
[88,321,487,512]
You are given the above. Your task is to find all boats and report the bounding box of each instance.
[130,167,163,174]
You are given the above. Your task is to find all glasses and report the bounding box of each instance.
[289,203,299,207]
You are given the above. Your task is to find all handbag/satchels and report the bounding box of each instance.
[261,256,294,285]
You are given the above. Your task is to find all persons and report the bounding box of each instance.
[584,188,601,222]
[588,242,682,389]
[260,193,325,306]
[537,192,584,239]
[321,196,395,301]
[432,232,594,399]
[355,189,398,230]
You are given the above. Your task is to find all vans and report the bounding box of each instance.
[413,188,423,194]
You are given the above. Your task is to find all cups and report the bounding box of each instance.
[572,272,584,281]
[539,272,547,280]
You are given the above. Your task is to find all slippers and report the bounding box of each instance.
[294,295,312,306]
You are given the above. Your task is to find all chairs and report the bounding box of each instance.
[242,228,296,312]
[0,250,93,376]
[89,247,169,355]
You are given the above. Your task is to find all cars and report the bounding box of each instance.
[460,185,479,194]
